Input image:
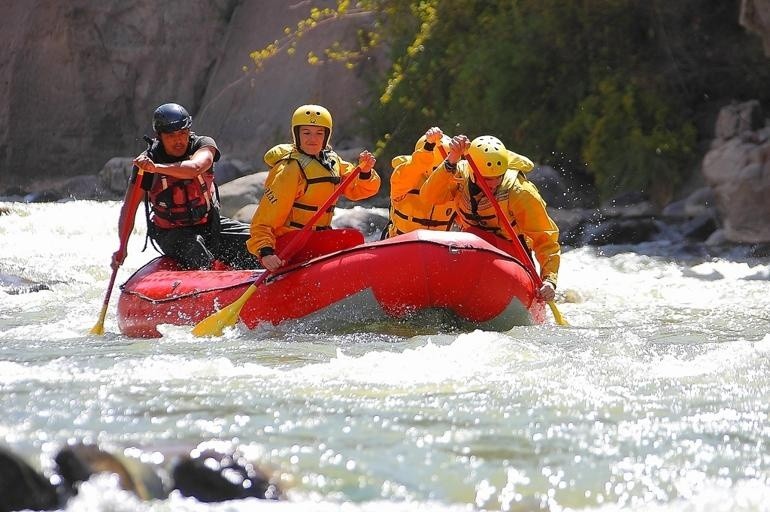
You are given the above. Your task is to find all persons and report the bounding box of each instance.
[420,134,561,303]
[380,126,462,241]
[110,103,266,271]
[245,105,381,273]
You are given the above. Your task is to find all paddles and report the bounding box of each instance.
[465,150,570,325]
[88,169,142,336]
[193,163,367,338]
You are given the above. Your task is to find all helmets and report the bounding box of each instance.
[292,105,333,148]
[154,103,192,133]
[468,136,508,183]
[415,132,453,178]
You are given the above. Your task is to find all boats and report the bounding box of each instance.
[116,223,548,339]
